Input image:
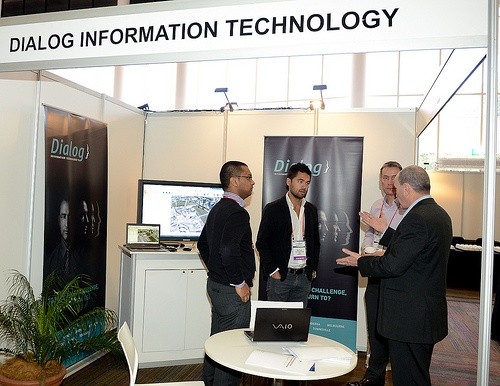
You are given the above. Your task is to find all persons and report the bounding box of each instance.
[256,163,321,308]
[51,199,95,283]
[347,161,407,386]
[318,208,353,245]
[336,165,452,386]
[197,161,256,386]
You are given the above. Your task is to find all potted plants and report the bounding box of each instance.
[0,270,122,386]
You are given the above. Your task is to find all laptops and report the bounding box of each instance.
[244,308,312,343]
[126,223,163,249]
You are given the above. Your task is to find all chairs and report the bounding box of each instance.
[451,237,464,245]
[118,320,205,386]
[476,238,500,246]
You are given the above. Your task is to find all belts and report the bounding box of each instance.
[288,268,306,274]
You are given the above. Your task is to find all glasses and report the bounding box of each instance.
[231,175,252,181]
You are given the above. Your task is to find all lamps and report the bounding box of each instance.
[215,88,234,112]
[313,84,327,110]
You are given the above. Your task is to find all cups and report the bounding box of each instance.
[364,247,375,254]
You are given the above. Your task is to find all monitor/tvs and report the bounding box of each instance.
[136,179,225,242]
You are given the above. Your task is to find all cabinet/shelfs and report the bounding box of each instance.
[118,245,213,368]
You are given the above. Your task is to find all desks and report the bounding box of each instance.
[446,245,500,291]
[205,326,358,386]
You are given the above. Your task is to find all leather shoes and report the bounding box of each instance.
[347,377,385,386]
